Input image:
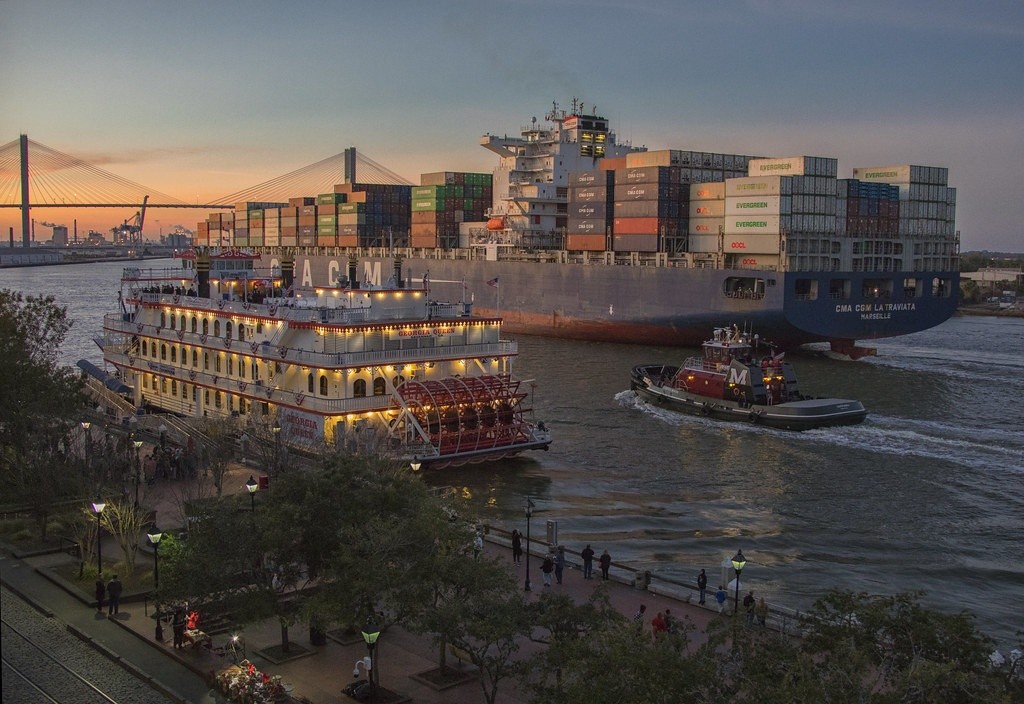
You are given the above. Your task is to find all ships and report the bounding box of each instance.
[175,96,962,358]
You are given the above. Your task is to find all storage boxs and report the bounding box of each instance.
[565,151,956,270]
[191,172,492,249]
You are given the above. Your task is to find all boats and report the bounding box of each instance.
[76,253,553,471]
[629,320,870,432]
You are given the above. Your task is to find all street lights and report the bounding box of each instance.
[133,433,144,508]
[91,493,106,573]
[731,549,747,655]
[147,522,163,641]
[272,420,281,460]
[82,417,91,465]
[361,615,381,704]
[521,496,535,590]
[245,475,257,512]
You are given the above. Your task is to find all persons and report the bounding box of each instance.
[442,508,484,562]
[634,603,678,643]
[168,601,199,649]
[715,585,768,626]
[581,544,595,579]
[96,572,123,614]
[143,282,196,296]
[143,432,209,484]
[599,549,611,582]
[512,529,522,566]
[540,549,564,586]
[697,569,707,606]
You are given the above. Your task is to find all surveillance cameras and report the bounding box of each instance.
[354,669,359,678]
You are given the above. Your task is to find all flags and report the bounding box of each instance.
[422,274,429,290]
[462,275,468,289]
[487,277,500,288]
